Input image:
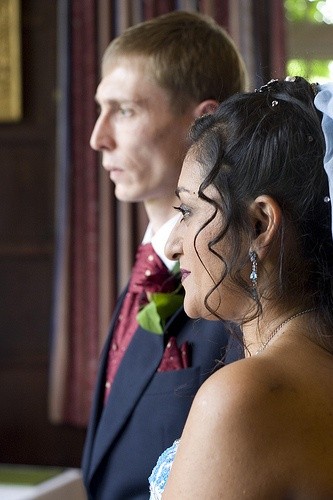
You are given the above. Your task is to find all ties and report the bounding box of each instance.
[97,242,176,409]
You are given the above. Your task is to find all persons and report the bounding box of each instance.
[145,76,332,500]
[78,10,249,500]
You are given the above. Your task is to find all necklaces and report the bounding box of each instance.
[253,306,322,356]
